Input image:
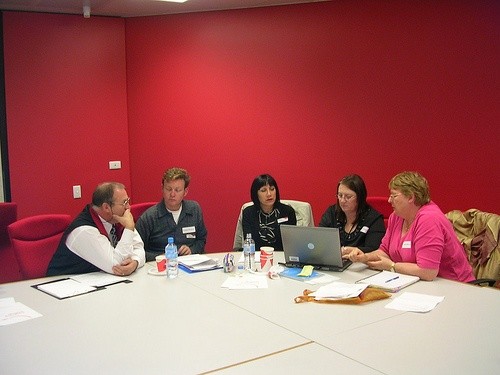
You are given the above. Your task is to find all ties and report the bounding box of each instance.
[110,224,118,248]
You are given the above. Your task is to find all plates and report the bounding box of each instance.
[148,268,166,275]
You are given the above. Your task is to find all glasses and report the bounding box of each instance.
[336,195,355,201]
[389,193,403,200]
[112,197,131,207]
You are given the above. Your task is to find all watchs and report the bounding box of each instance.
[391,262,395,272]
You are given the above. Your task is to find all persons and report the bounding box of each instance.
[242,174,296,252]
[46,182,146,276]
[348,172,476,285]
[318,174,385,260]
[136,167,206,263]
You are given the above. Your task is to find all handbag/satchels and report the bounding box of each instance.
[294,286,390,304]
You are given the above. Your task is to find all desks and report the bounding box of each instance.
[0,250,500,374]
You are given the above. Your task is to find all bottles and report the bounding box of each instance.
[165,238,178,278]
[243,233,255,269]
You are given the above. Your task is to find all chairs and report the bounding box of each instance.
[6,213,72,280]
[365,196,395,232]
[232,200,315,252]
[0,201,21,284]
[116,201,158,241]
[444,209,500,291]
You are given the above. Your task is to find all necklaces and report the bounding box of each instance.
[406,222,410,230]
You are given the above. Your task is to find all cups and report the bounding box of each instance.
[155,255,166,272]
[260,247,274,269]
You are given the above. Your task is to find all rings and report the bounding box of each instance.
[123,273,125,275]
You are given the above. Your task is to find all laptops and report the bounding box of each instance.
[280,225,352,271]
